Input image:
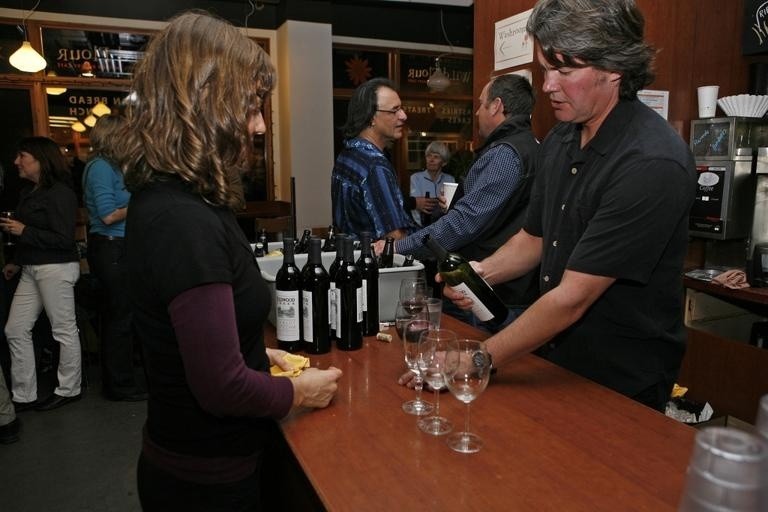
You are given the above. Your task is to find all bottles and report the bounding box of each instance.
[356,232,380,337]
[335,238,363,351]
[421,234,509,329]
[253,242,263,257]
[301,235,318,273]
[402,254,414,267]
[259,228,268,254]
[323,225,334,248]
[300,230,311,247]
[300,238,331,355]
[423,191,431,225]
[381,237,395,268]
[328,233,346,341]
[275,237,300,353]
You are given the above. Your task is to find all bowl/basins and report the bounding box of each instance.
[716,94,768,117]
[250,241,376,258]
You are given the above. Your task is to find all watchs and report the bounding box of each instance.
[471,343,497,377]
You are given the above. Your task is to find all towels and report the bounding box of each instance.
[711,268,751,290]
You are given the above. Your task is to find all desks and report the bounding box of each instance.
[260,310,699,511]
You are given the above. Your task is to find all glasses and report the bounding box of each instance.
[377,108,401,116]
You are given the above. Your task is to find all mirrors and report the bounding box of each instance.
[28,77,269,164]
[1,16,271,88]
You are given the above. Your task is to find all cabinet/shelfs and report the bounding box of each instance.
[683,273,768,419]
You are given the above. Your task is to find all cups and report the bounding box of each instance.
[416,328,460,436]
[425,298,443,331]
[402,319,435,416]
[678,426,768,512]
[688,267,706,279]
[395,299,431,384]
[1,212,16,245]
[399,278,427,317]
[444,339,490,454]
[705,262,721,278]
[443,182,459,210]
[697,85,720,118]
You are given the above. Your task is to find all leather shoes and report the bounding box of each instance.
[108,387,151,402]
[13,397,38,414]
[34,391,84,412]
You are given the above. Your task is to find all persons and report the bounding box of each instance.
[0,361,24,442]
[404,196,438,211]
[0,137,83,411]
[109,16,344,512]
[371,73,540,261]
[331,76,420,241]
[85,113,152,402]
[399,1,696,410]
[409,141,458,214]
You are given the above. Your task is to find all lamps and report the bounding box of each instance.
[8,0,47,73]
[426,9,455,89]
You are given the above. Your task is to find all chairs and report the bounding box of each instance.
[255,214,290,235]
